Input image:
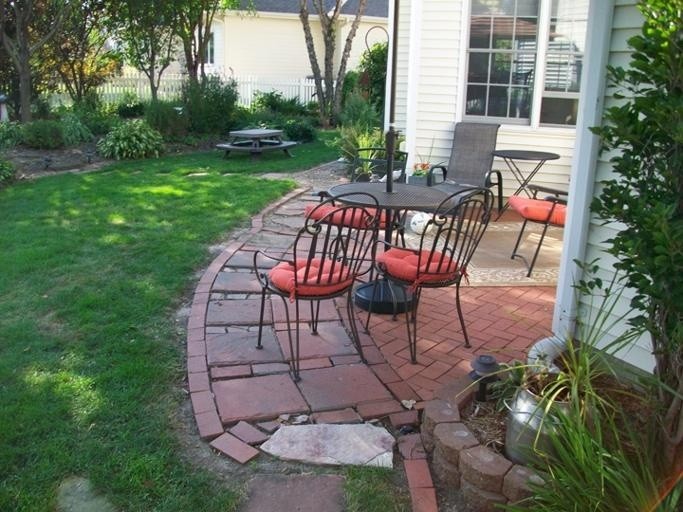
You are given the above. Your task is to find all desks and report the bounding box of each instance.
[223,128,292,161]
[493,150,559,220]
[329,181,453,314]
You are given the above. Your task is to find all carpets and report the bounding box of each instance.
[429,218,566,286]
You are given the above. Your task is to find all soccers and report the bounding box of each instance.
[410,212,433,235]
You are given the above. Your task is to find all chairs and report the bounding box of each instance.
[508,185,571,278]
[306,148,407,257]
[253,192,380,382]
[428,123,504,215]
[363,186,495,365]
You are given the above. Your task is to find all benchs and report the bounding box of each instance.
[216,140,296,152]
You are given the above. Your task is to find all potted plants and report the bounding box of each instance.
[502,268,646,471]
